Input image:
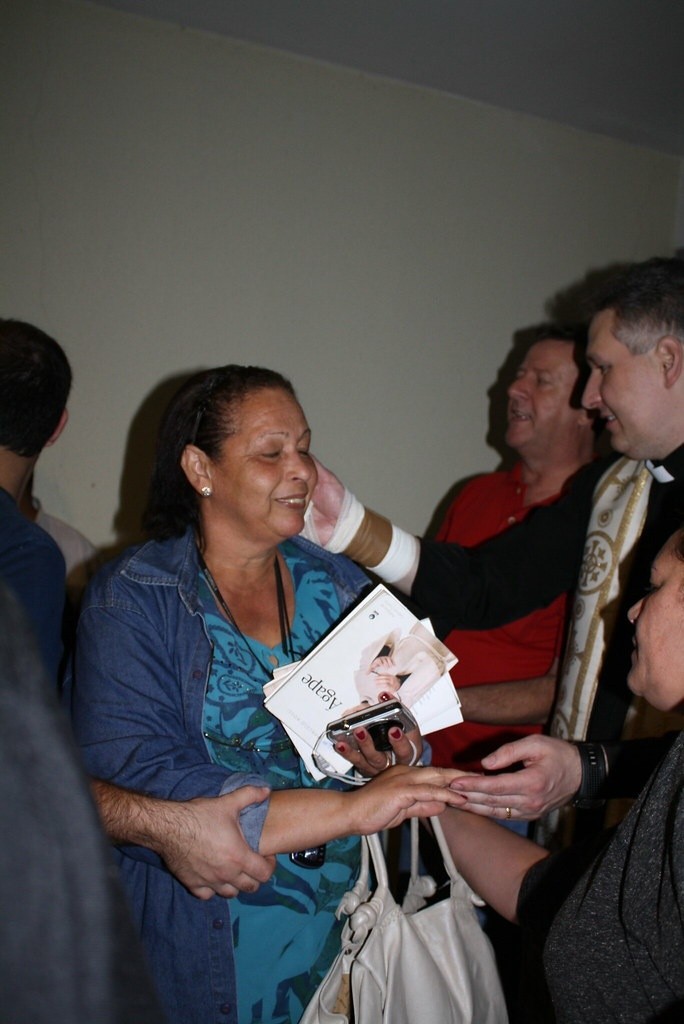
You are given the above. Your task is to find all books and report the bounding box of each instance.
[262,583,464,781]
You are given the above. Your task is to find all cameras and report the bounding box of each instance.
[327,699,418,751]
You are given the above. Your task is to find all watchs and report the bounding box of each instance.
[570,741,607,810]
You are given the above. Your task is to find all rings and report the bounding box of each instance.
[506,807,511,820]
[380,756,389,770]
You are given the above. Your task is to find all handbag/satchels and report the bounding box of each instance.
[297,813,509,1024]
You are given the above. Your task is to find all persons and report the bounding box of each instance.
[0,581,163,1024]
[19,470,97,599]
[0,319,277,901]
[422,528,684,1024]
[296,258,684,849]
[398,322,609,928]
[340,620,456,718]
[73,365,482,1024]
